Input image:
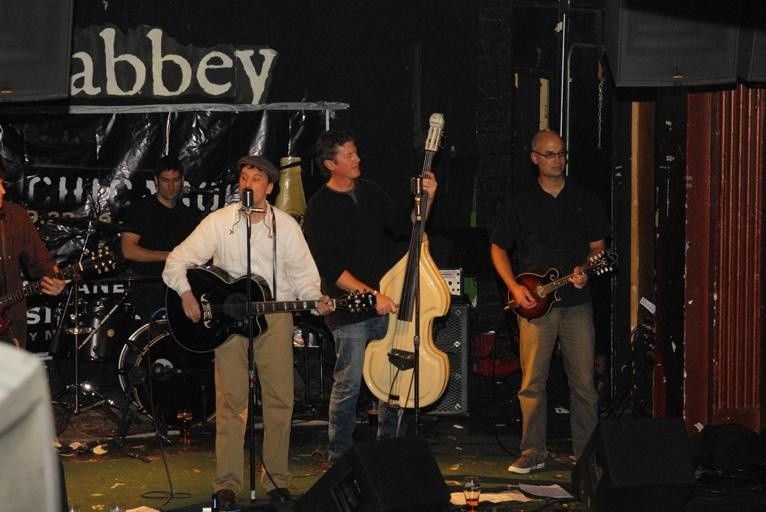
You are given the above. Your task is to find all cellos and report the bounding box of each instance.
[363,114,450,408]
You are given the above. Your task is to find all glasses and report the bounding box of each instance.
[531,148,567,160]
[156,175,181,183]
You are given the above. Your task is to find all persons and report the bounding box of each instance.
[0,168,72,348]
[490,129,612,474]
[303,129,437,472]
[161,155,335,512]
[121,155,194,324]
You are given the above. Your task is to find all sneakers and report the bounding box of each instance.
[566,454,578,469]
[267,486,296,502]
[376,429,393,440]
[507,447,545,475]
[212,488,241,512]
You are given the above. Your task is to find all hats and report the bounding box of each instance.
[235,155,280,183]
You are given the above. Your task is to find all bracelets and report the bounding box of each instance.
[372,291,380,296]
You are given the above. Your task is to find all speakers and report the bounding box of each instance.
[740,7,765,83]
[598,3,739,88]
[294,433,450,512]
[570,417,704,512]
[0,0,74,102]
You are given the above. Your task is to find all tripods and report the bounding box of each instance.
[50,271,142,433]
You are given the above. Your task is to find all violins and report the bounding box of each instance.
[505,249,620,322]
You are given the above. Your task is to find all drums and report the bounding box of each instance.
[118,320,214,428]
[70,312,127,362]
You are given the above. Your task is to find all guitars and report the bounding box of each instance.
[164,265,377,353]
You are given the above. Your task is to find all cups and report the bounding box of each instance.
[108,502,126,511]
[67,504,81,512]
[462,477,481,511]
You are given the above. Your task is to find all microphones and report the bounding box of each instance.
[413,176,423,216]
[86,221,96,235]
[242,188,253,215]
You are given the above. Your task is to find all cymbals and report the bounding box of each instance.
[97,274,158,284]
[52,216,120,236]
